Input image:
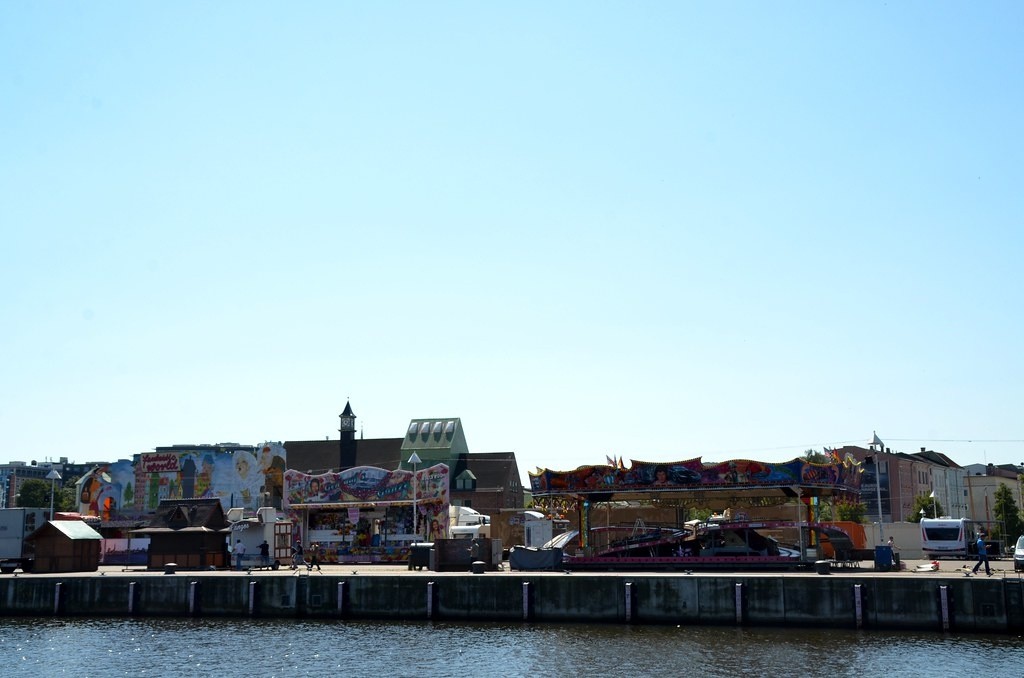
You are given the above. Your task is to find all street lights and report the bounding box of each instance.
[929,491,939,519]
[406,451,423,543]
[866,431,886,546]
[46,468,62,521]
[919,508,926,518]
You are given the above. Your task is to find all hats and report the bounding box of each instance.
[472,538,478,542]
[297,540,300,542]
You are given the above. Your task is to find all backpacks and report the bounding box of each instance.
[972,540,981,553]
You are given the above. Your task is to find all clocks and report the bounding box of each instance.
[351,420,353,426]
[343,419,349,425]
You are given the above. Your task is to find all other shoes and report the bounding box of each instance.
[318,568,320,570]
[290,568,295,570]
[307,567,312,571]
[972,570,977,575]
[987,572,992,575]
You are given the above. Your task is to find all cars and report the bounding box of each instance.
[1010,534,1024,573]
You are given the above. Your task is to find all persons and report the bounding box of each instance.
[650,465,670,484]
[216,452,260,512]
[230,539,246,570]
[302,479,331,503]
[467,538,479,572]
[888,536,902,564]
[429,520,445,541]
[291,539,321,571]
[256,540,271,571]
[972,533,994,577]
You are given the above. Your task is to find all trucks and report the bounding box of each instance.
[0,506,102,573]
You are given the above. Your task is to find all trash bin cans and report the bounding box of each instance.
[411,542,434,568]
